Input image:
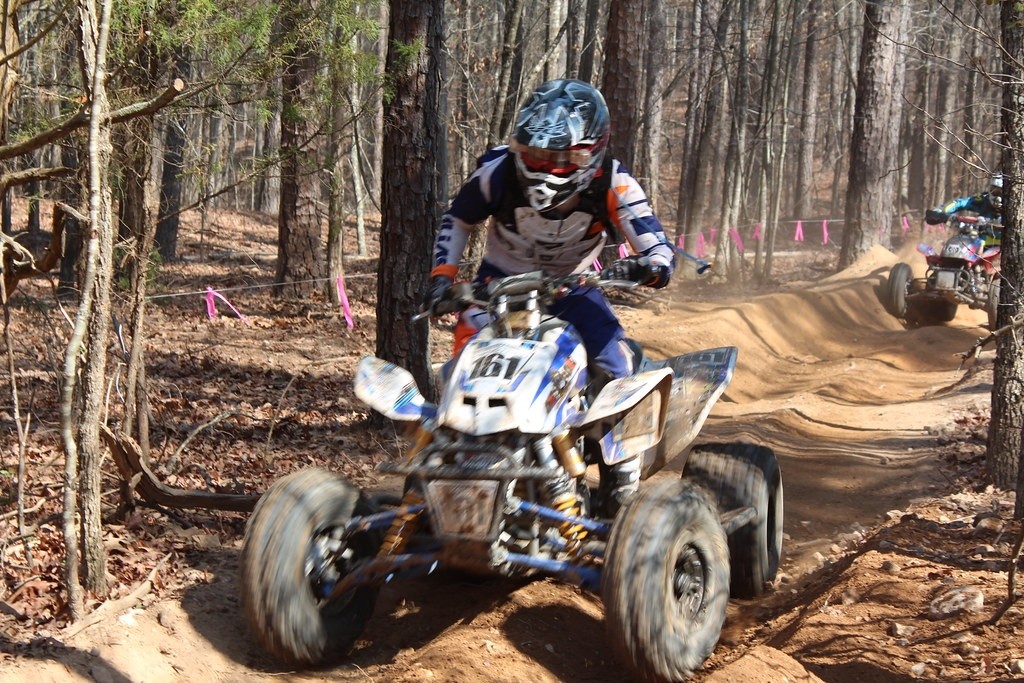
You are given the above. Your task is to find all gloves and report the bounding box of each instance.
[606,253,653,284]
[423,275,452,318]
[925,205,948,225]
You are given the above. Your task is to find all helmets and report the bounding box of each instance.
[509,79,611,213]
[989,175,1005,209]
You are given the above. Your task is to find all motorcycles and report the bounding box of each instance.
[887,209,1002,331]
[241,258,784,683]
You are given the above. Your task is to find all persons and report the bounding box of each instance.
[423,78,679,542]
[924,171,1005,300]
[55,129,86,298]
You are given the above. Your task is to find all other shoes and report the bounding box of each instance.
[595,460,642,516]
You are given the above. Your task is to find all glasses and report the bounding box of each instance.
[520,147,580,175]
